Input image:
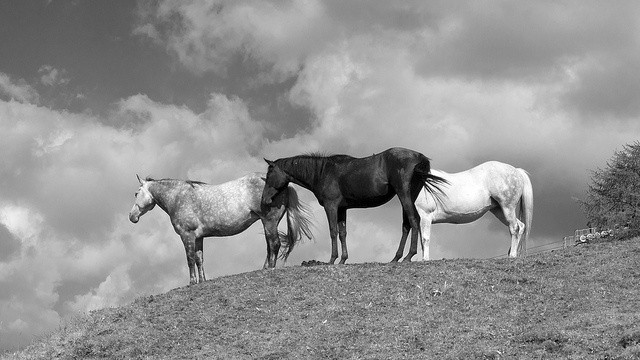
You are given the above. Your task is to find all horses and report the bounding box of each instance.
[412,159,534,261]
[260,145,450,264]
[128,173,313,285]
